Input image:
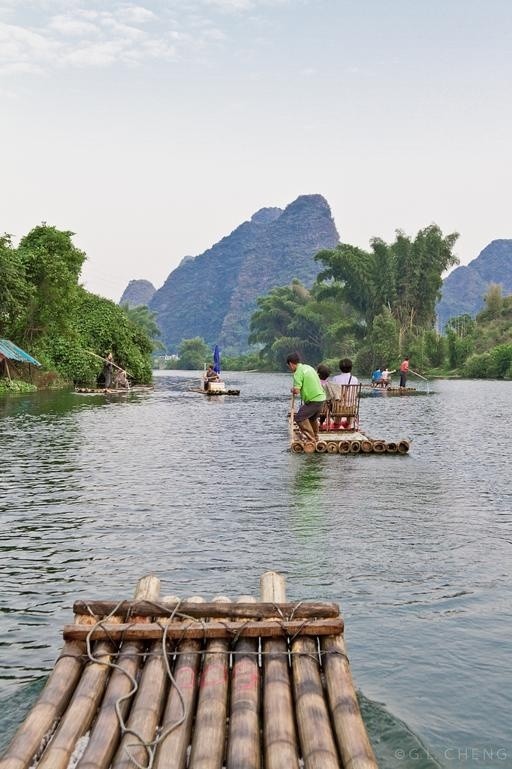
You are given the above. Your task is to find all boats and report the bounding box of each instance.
[362,387,433,395]
[191,386,240,396]
[0,572,378,769]
[75,384,152,395]
[288,411,411,455]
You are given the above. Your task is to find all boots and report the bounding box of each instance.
[298,419,319,443]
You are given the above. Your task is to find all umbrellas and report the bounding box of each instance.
[214,345,220,380]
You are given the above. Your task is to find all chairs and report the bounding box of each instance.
[328,383,362,433]
[320,401,328,432]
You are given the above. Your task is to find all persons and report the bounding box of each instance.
[331,358,358,428]
[204,365,219,391]
[372,368,384,388]
[287,353,326,445]
[382,368,396,387]
[96,352,114,388]
[316,364,340,431]
[114,369,129,389]
[399,358,409,388]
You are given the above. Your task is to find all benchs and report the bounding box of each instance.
[375,378,391,388]
